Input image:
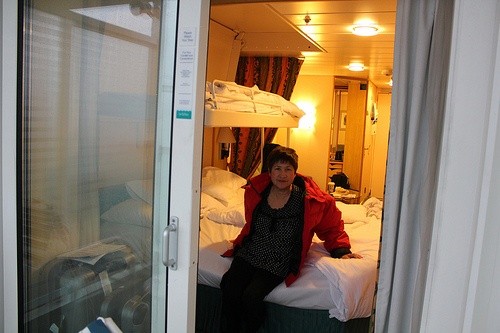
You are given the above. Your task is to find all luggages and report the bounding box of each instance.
[40,240,151,333]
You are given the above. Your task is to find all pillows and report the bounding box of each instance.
[101,198,151,229]
[200,166,247,203]
[125,180,221,210]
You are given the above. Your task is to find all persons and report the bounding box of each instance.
[219,146,362,333]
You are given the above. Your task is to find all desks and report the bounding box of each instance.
[330,187,359,204]
[328,162,343,175]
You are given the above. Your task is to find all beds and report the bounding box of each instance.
[113,201,380,323]
[204,79,299,174]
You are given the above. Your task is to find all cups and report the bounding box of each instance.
[328,182,335,192]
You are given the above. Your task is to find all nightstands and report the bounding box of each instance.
[49,247,152,333]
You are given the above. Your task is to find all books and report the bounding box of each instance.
[55,241,128,266]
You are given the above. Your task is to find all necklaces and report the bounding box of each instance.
[274,195,287,203]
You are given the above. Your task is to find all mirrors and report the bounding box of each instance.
[325,76,369,204]
